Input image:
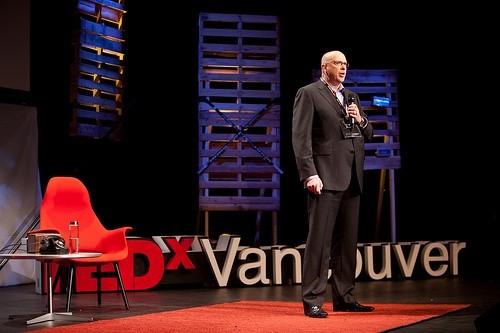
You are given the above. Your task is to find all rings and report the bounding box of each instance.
[356,111,357,115]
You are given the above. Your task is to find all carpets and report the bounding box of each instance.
[40,177,133,312]
[14,299,471,333]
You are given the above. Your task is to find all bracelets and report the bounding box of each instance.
[359,118,365,127]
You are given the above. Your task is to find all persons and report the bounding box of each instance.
[292,50,375,319]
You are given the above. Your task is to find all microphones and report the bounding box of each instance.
[347,94,355,130]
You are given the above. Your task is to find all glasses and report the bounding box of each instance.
[326,61,350,68]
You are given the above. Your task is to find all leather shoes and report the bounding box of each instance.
[334,301,374,312]
[304,305,328,318]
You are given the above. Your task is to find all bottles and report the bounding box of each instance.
[68,221,79,254]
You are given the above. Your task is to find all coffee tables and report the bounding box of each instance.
[0,250,102,325]
